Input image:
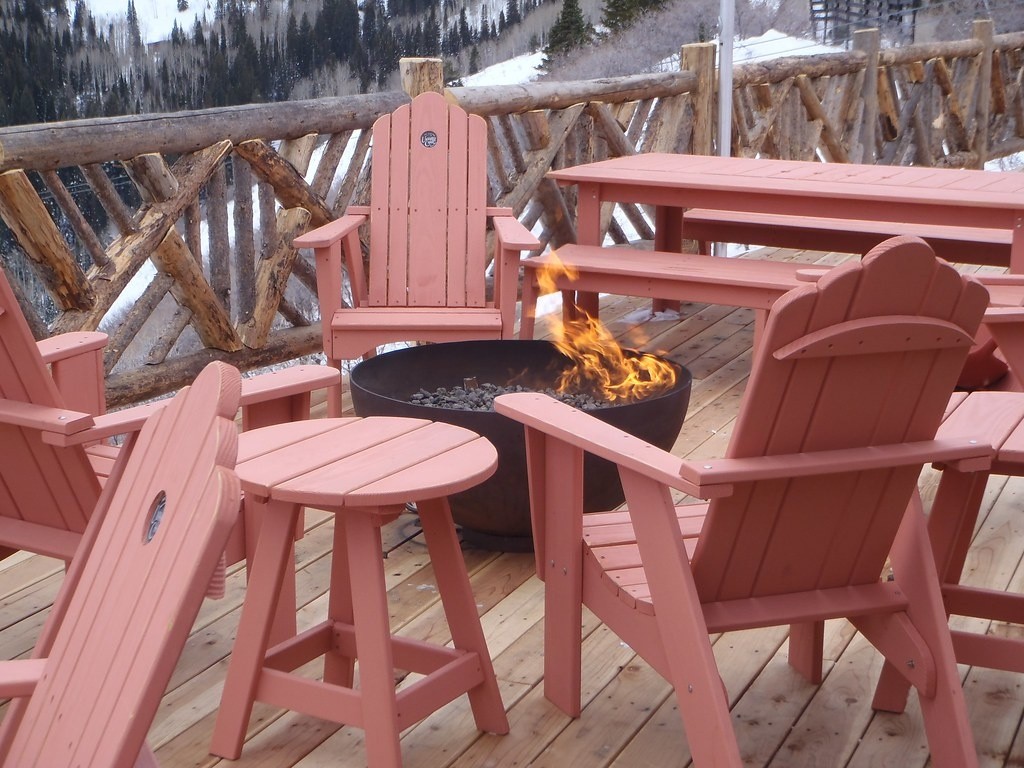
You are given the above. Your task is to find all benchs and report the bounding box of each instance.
[682,209,1013,267]
[515,243,1024,341]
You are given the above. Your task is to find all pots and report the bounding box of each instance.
[350,339,692,536]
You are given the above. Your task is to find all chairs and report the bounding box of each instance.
[0,359,242,768]
[870,391,1024,714]
[0,266,341,573]
[493,234,990,768]
[292,91,541,416]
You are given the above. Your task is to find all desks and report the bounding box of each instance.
[542,152,1024,332]
[208,417,509,768]
